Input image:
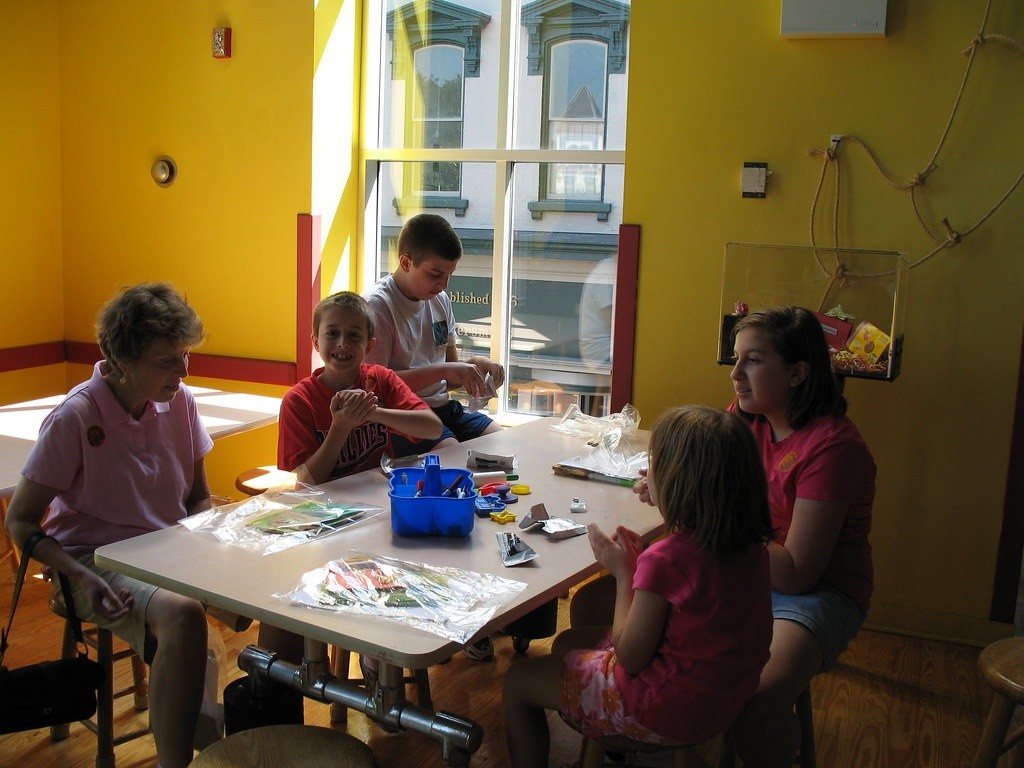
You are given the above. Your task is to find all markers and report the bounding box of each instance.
[441,475,465,497]
[457,485,467,498]
[413,480,423,498]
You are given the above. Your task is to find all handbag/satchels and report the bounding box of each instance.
[0,532,98,735]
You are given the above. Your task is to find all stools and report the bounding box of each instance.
[47,466,378,768]
[969,636,1024,768]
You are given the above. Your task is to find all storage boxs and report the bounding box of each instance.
[389,454,478,538]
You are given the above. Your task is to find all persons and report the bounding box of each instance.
[577,249,616,369]
[5,280,304,768]
[363,214,504,455]
[571,307,877,768]
[502,404,774,768]
[278,291,495,697]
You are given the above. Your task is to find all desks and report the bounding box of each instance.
[93,417,670,768]
[0,386,284,580]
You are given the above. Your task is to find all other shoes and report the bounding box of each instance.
[463,634,494,660]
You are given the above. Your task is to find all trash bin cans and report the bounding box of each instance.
[224,676,304,737]
